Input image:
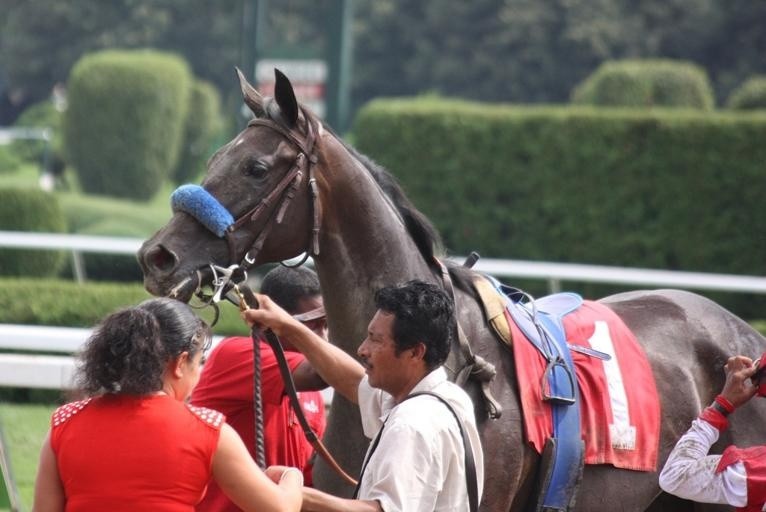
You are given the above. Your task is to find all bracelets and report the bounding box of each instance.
[712,401,730,416]
[279,466,298,483]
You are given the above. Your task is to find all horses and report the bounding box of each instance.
[131,63,765,512]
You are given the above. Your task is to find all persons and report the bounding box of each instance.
[188,262,333,512]
[658,348,766,512]
[29,293,306,511]
[239,276,486,512]
